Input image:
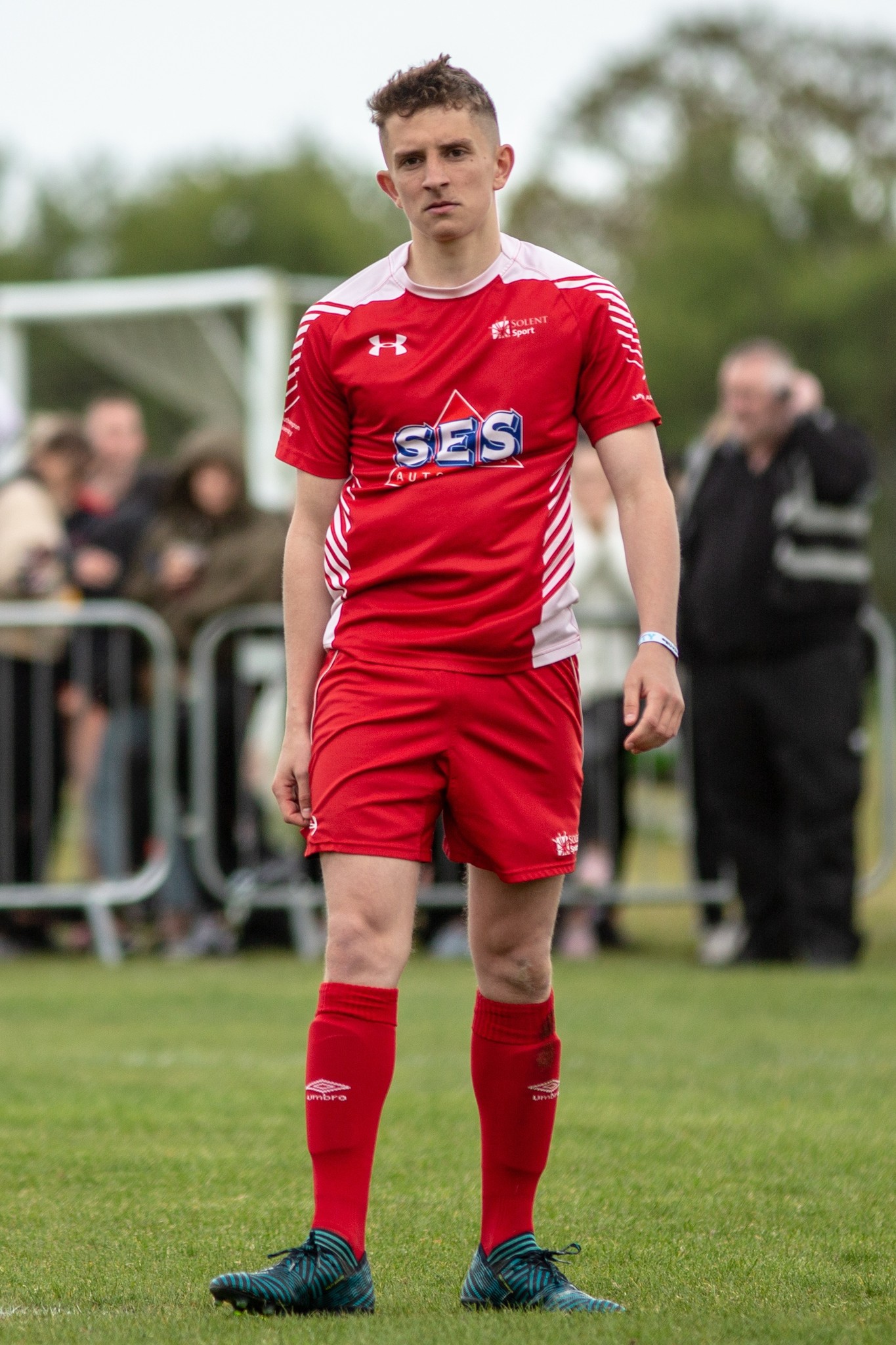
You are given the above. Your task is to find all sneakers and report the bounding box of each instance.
[458,1230,626,1314]
[210,1227,375,1316]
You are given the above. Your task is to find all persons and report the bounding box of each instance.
[0,325,878,968]
[211,55,687,1317]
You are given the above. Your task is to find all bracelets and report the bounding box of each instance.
[639,632,682,660]
